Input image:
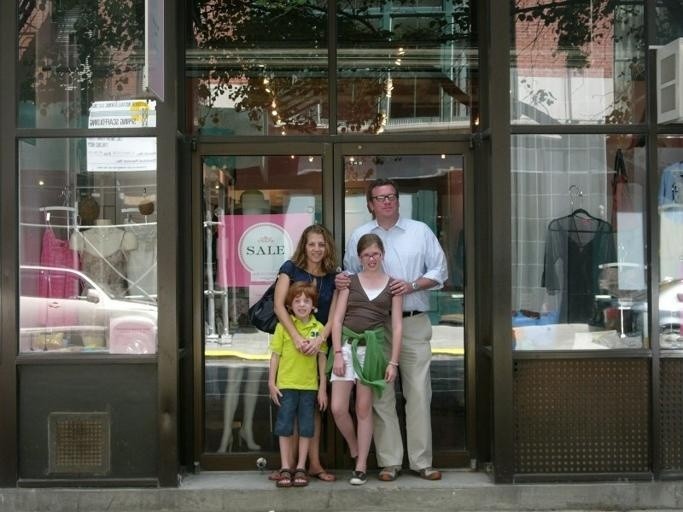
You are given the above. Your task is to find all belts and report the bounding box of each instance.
[389,311,422,317]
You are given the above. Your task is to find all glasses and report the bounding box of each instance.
[371,194,397,202]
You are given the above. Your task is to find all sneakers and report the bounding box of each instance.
[408,467,441,480]
[378,465,402,480]
[350,456,368,485]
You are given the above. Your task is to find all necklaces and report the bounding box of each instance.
[306,270,321,314]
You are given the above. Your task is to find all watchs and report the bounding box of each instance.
[409,280,419,292]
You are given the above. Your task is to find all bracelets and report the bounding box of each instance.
[319,333,326,343]
[332,349,342,355]
[387,358,400,368]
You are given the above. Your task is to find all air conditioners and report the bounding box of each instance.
[648,37,683,125]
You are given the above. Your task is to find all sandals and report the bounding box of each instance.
[269,464,336,488]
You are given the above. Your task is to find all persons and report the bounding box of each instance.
[334,179,448,480]
[266,277,328,490]
[216,182,271,456]
[330,231,402,486]
[67,215,139,351]
[268,224,336,482]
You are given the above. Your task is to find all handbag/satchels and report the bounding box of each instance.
[249,261,295,334]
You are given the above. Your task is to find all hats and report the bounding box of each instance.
[236,190,270,209]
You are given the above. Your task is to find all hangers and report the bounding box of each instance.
[122,190,159,227]
[548,190,613,233]
[38,185,80,216]
